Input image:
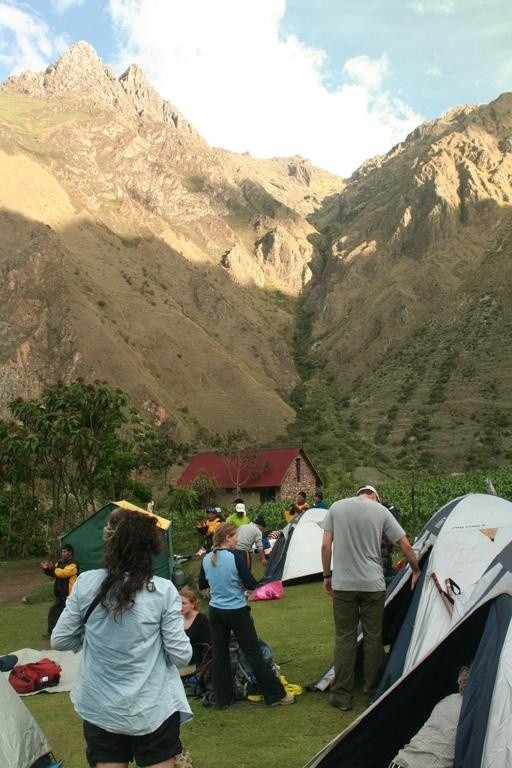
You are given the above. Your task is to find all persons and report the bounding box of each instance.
[41,543,79,641]
[197,523,295,713]
[196,492,332,590]
[178,589,212,685]
[50,507,195,768]
[328,483,422,712]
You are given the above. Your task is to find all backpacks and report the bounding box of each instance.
[8,658,62,693]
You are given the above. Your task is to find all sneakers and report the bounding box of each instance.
[264,694,297,707]
[328,691,354,712]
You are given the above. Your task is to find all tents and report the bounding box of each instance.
[0,668,65,768]
[303,492,512,768]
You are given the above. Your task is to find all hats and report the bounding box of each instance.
[235,502,245,512]
[254,515,266,528]
[355,485,381,503]
[315,492,323,500]
[206,508,217,514]
